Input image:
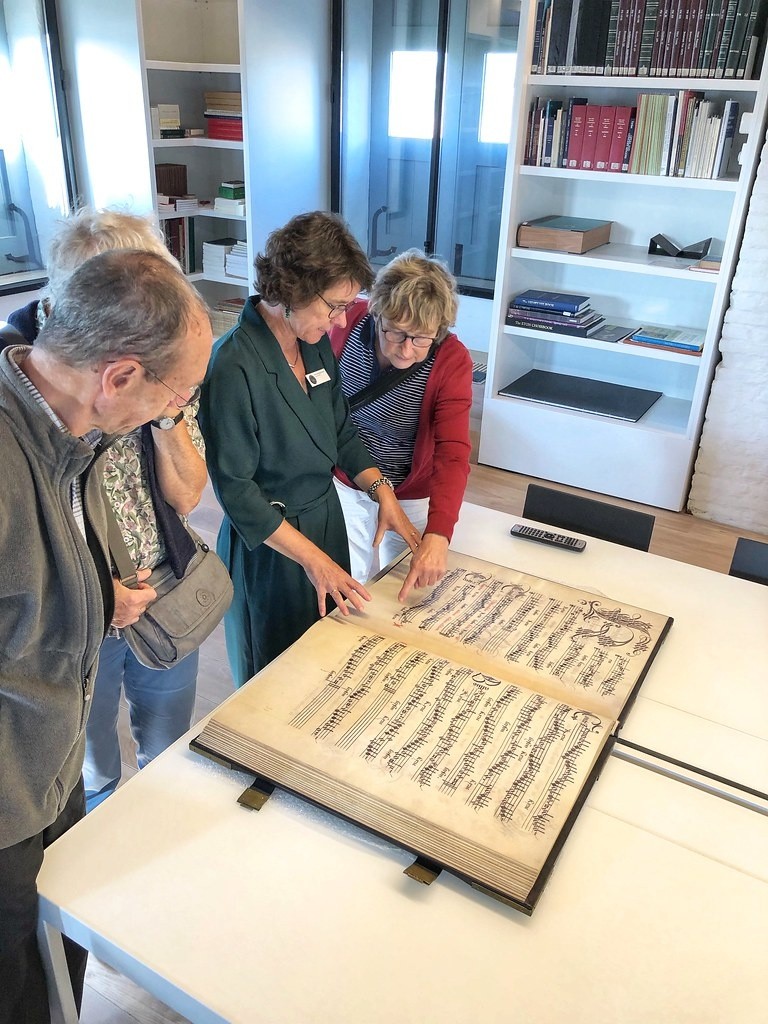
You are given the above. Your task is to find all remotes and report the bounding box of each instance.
[510,524,586,552]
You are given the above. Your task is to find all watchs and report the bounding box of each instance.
[151,410,183,431]
[367,476,394,503]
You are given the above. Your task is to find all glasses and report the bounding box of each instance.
[106,360,202,407]
[316,293,357,319]
[379,316,440,348]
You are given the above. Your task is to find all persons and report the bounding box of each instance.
[325,247,472,602]
[5,206,207,814]
[0,248,211,1024]
[196,209,422,691]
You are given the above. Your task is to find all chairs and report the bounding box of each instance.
[523,484,655,552]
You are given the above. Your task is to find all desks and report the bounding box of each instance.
[33,501,768,1024]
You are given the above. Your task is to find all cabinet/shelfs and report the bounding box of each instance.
[56,0,335,290]
[477,0,768,514]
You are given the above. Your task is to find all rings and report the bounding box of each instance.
[255,304,299,368]
[138,609,143,616]
[330,588,339,595]
[410,532,416,536]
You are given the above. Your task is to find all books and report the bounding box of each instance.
[209,298,247,338]
[214,180,245,217]
[202,237,248,279]
[159,217,195,275]
[531,0,768,81]
[506,289,607,338]
[150,90,243,141]
[497,368,663,422]
[191,541,674,916]
[617,326,706,356]
[689,255,722,274]
[155,163,199,212]
[523,90,739,180]
[516,214,614,255]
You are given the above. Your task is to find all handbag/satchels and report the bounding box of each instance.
[121,542,233,671]
